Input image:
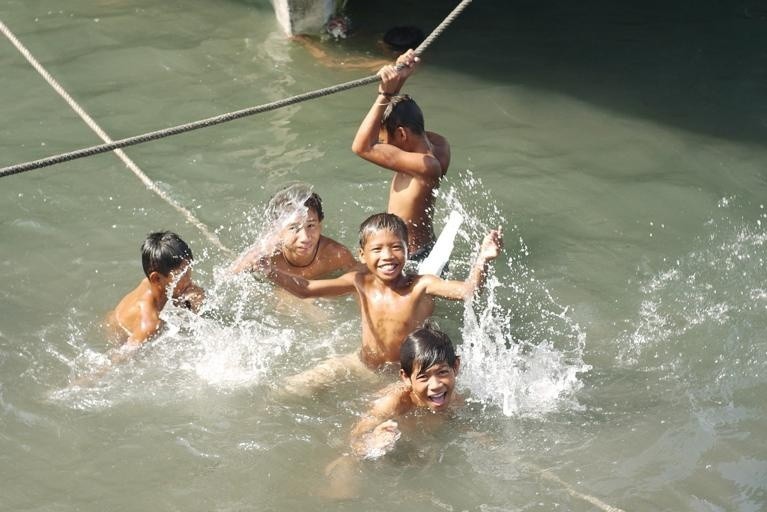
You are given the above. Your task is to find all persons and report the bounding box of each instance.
[252,217,505,411]
[103,228,204,362]
[351,49,450,278]
[328,320,466,438]
[229,184,354,319]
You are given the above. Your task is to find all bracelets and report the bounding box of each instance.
[378,91,393,97]
